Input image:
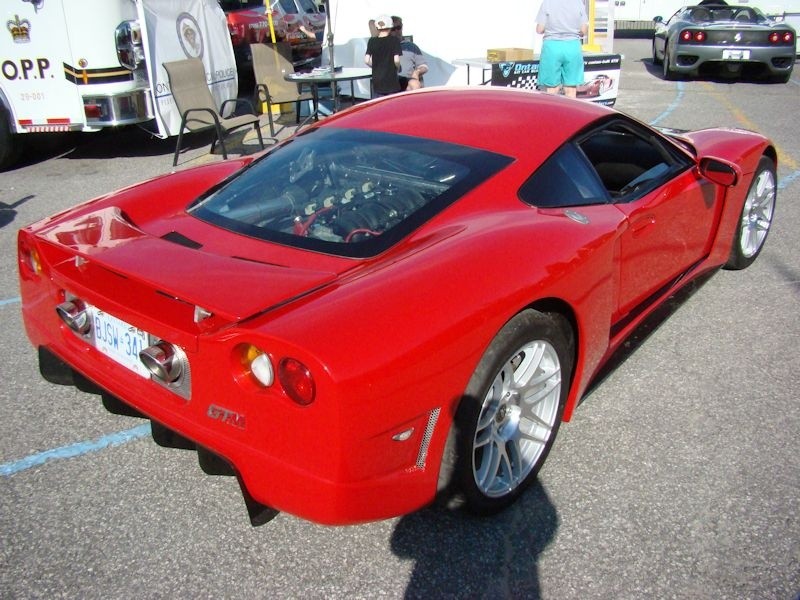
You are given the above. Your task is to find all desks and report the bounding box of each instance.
[450,49,624,87]
[283,65,374,124]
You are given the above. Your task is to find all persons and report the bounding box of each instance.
[387,17,429,92]
[364,16,402,98]
[536,0,590,100]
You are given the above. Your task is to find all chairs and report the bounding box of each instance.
[249,39,321,135]
[161,58,267,169]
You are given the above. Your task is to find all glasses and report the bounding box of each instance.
[391,24,402,31]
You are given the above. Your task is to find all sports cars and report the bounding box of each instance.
[652,0,797,86]
[18,86,779,525]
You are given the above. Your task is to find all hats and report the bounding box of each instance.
[375,17,394,28]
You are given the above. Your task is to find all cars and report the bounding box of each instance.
[0,2,326,162]
